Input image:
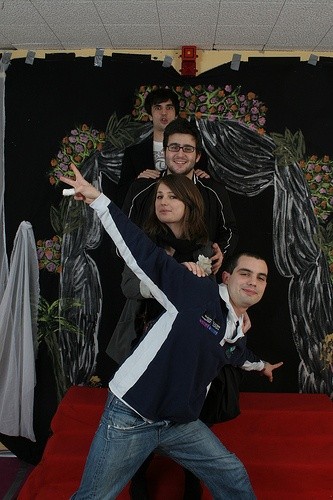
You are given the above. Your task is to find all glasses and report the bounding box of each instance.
[165,144,197,153]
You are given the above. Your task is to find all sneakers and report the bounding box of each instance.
[84,375,111,388]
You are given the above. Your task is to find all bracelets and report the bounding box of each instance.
[262,361,266,372]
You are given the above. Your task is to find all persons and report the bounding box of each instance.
[58,163,284,500]
[105,175,216,500]
[118,88,207,207]
[116,117,238,276]
